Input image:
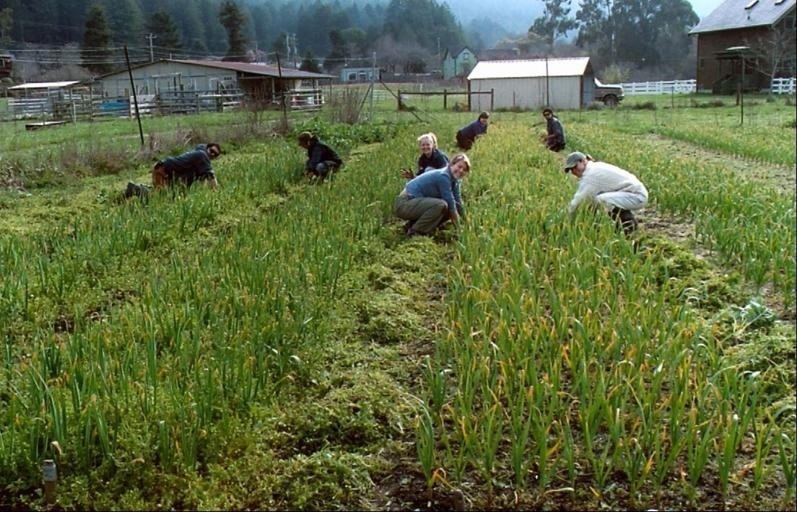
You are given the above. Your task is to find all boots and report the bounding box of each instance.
[608,207,638,236]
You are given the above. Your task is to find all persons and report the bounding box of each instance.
[297,131,342,184]
[456,111,489,150]
[125,143,221,200]
[549,152,649,235]
[392,153,471,238]
[539,109,565,152]
[399,132,450,180]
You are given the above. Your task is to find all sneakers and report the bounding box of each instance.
[125,182,135,198]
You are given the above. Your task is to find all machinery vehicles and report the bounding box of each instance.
[1,54,25,97]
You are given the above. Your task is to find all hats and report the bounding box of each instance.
[564,151,587,173]
[298,131,312,146]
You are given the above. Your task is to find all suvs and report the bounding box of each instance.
[593,77,625,108]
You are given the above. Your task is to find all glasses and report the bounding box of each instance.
[209,149,219,157]
